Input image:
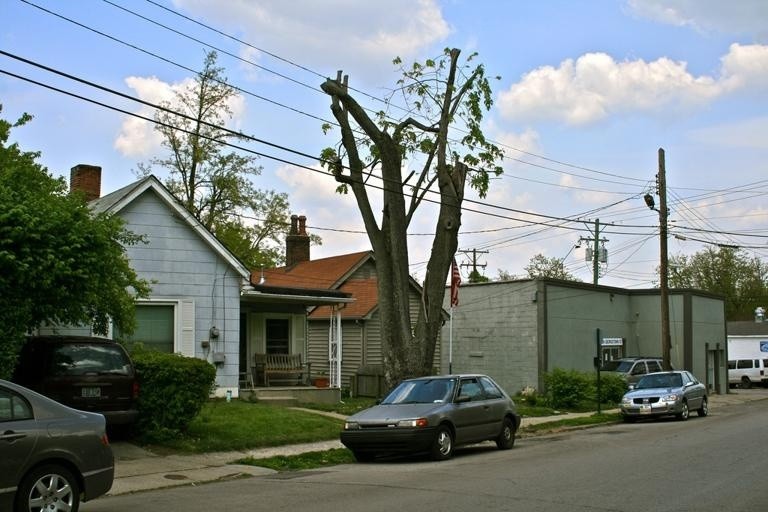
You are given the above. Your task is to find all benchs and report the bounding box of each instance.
[254,353,313,387]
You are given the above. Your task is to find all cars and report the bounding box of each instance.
[0,378,114,512]
[341,374,521,462]
[12,335,141,438]
[620,370,708,420]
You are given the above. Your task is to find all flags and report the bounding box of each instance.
[452,257,462,308]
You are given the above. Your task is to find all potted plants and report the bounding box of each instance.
[313,371,329,387]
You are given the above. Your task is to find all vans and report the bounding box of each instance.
[728,358,768,389]
[600,356,675,389]
